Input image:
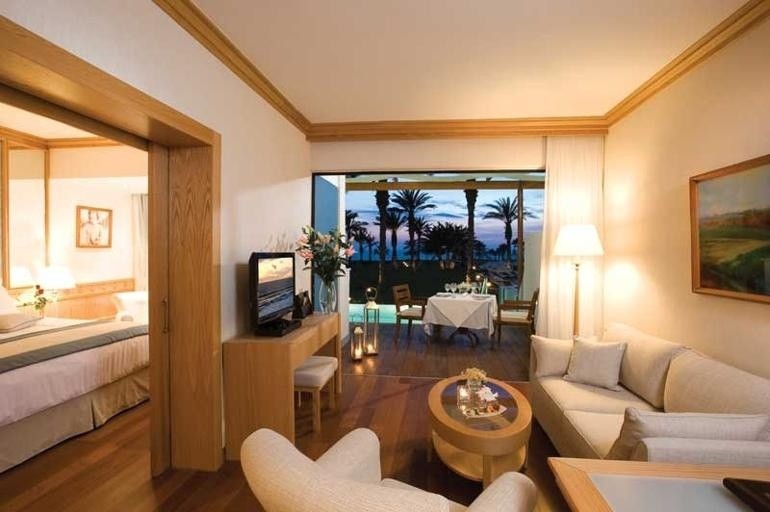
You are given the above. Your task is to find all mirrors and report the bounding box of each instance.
[1,143,50,289]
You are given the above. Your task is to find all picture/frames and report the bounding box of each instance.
[75,206,113,249]
[688,154,770,305]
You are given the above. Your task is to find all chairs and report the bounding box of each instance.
[392,284,427,337]
[496,288,540,343]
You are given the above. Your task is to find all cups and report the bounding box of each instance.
[445,283,451,296]
[470,282,477,296]
[476,400,485,416]
[457,384,472,411]
[449,282,457,297]
[488,398,500,413]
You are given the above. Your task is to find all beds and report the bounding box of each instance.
[0,317,148,476]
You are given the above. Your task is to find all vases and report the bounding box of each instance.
[468,378,483,390]
[319,279,337,314]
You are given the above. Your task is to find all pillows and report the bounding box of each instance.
[562,335,626,393]
[530,334,598,378]
[0,313,44,334]
[603,407,768,459]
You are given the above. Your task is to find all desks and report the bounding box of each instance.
[549,454,768,512]
[222,311,343,461]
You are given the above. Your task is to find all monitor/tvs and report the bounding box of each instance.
[246,251,301,338]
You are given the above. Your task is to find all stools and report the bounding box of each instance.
[294,356,338,431]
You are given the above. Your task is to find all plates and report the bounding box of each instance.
[459,404,508,419]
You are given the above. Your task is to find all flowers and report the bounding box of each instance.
[460,366,487,382]
[295,224,359,284]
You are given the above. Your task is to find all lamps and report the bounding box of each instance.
[9,266,36,288]
[551,223,604,336]
[40,265,76,301]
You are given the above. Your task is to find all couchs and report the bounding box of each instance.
[529,323,770,468]
[242,426,538,511]
